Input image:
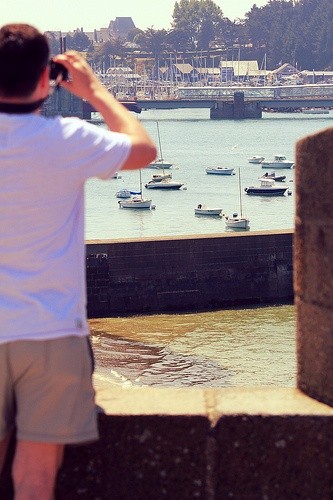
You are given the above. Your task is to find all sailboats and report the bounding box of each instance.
[225,167,250,229]
[143,120,185,190]
[118,167,153,209]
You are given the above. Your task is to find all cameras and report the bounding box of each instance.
[47,61,67,81]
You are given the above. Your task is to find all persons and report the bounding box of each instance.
[0,23,158,500]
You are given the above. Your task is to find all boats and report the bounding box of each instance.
[262,154,295,169]
[244,178,289,196]
[257,170,286,182]
[194,203,224,216]
[147,159,175,169]
[113,172,123,180]
[247,156,265,164]
[114,188,141,199]
[205,166,235,175]
